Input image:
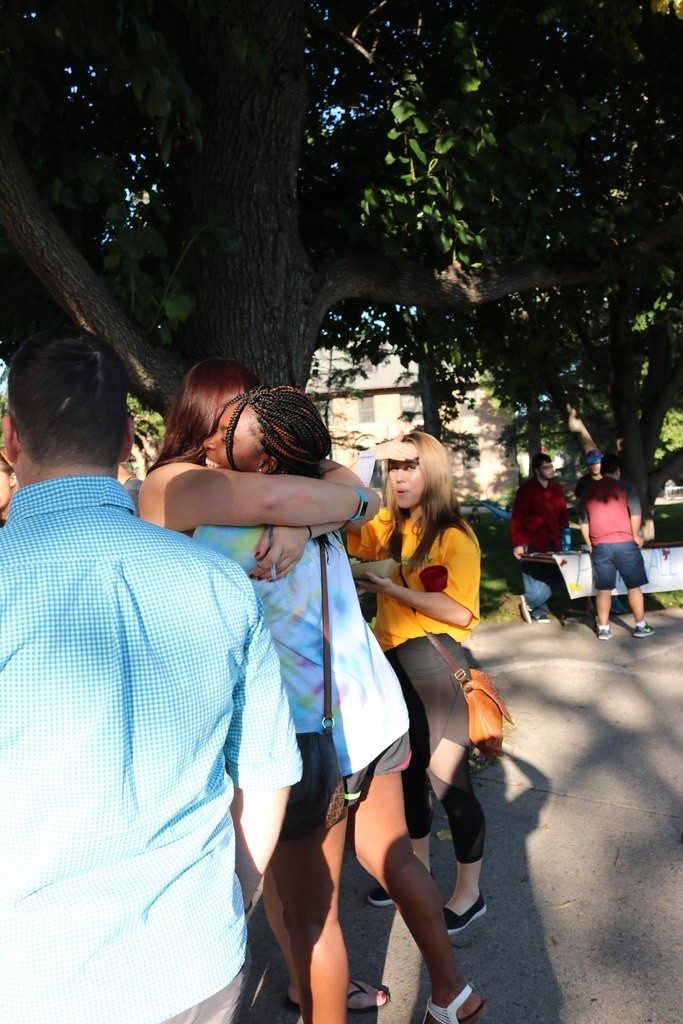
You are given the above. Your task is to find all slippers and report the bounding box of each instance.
[286,982,389,1012]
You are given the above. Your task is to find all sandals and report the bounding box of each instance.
[423,983,489,1024]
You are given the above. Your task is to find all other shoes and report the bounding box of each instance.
[366,869,432,907]
[441,893,487,935]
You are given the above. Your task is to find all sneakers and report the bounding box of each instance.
[597,627,613,639]
[632,622,655,639]
[536,613,550,623]
[520,594,533,622]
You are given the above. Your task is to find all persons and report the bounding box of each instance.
[137,355,388,1013]
[1,323,302,1023]
[577,454,655,641]
[189,385,485,1023]
[345,430,490,936]
[468,507,482,524]
[510,453,569,624]
[573,449,604,507]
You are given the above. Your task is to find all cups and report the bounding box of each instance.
[419,565,448,593]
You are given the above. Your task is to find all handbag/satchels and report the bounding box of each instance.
[463,667,515,760]
[277,731,345,840]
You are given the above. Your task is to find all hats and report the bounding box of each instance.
[586,450,601,464]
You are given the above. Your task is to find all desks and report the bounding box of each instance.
[518,542,683,626]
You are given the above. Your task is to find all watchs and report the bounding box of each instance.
[351,486,368,521]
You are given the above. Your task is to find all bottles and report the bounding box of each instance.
[562,528,572,551]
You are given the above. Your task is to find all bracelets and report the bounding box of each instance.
[305,525,312,541]
